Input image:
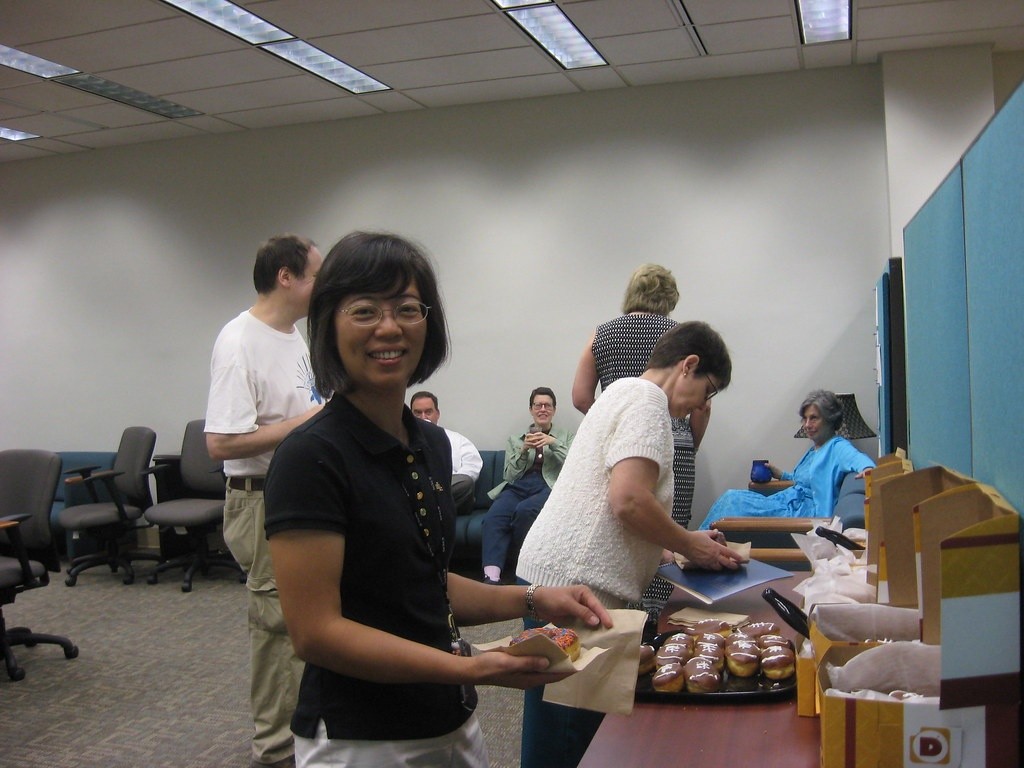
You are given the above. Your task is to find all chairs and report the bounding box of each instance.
[59,426,166,587]
[0,448,78,681]
[144,419,247,592]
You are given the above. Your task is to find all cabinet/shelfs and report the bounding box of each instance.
[872,258,908,461]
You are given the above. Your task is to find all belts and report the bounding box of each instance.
[226,477,264,491]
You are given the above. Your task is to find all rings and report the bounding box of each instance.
[716,567,724,574]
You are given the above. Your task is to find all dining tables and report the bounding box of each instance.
[578,570,821,767]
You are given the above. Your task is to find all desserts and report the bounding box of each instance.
[636,618,795,691]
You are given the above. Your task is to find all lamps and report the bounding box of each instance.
[792,392,876,441]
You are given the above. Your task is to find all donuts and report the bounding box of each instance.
[509,627,581,663]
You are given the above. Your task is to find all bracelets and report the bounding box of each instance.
[524,584,543,622]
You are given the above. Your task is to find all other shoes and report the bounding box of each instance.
[477,573,502,585]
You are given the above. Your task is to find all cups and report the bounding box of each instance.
[750,460,772,482]
[529,427,542,449]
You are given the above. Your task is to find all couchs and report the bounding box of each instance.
[50,451,119,560]
[466,450,506,568]
[709,493,866,572]
[718,472,865,521]
[449,451,496,569]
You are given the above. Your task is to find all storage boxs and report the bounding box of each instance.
[810,467,981,717]
[841,446,906,559]
[794,460,915,717]
[816,483,1022,768]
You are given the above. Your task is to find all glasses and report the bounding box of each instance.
[705,374,718,400]
[336,302,432,326]
[534,402,553,410]
[801,417,824,426]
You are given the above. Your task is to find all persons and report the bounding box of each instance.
[696,389,877,530]
[259,229,613,768]
[474,386,574,585]
[410,391,482,517]
[204,235,328,768]
[570,263,712,617]
[514,320,746,768]
